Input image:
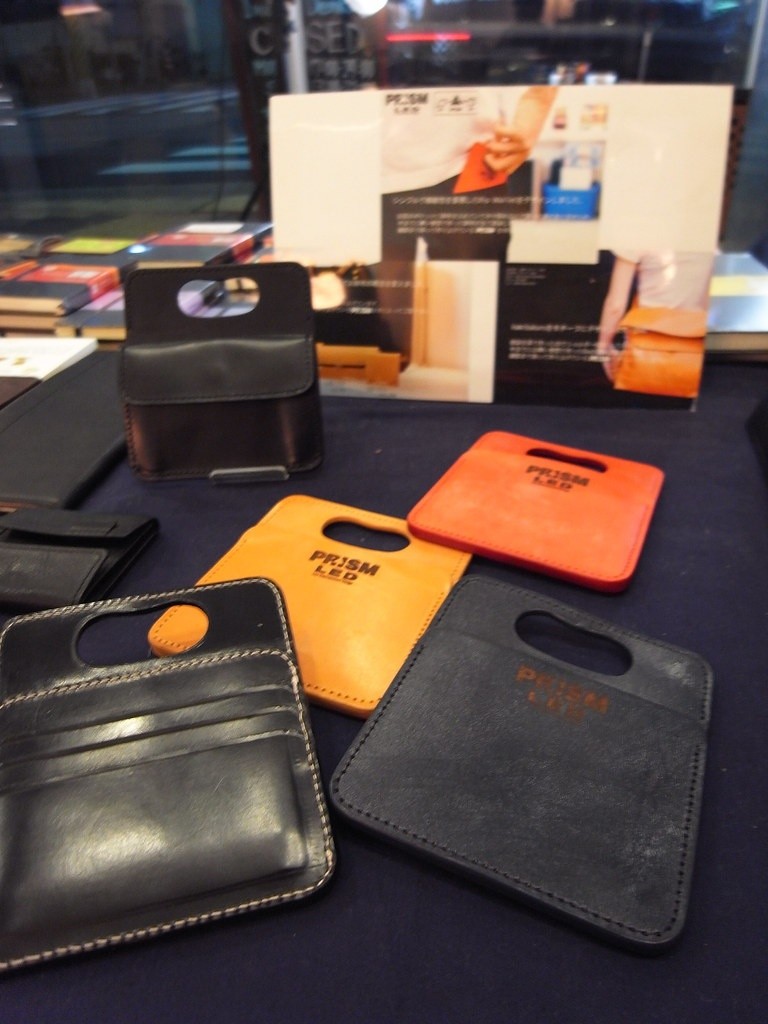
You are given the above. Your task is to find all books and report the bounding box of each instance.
[0,212,277,516]
[704,251,767,364]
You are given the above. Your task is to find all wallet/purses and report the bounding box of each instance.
[0,502,160,611]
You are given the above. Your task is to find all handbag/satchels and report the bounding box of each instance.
[612,299,706,396]
[0,577,336,974]
[324,577,716,945]
[404,432,668,592]
[147,492,475,716]
[114,257,326,479]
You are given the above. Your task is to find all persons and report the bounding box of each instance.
[372,87,563,260]
[597,248,711,385]
